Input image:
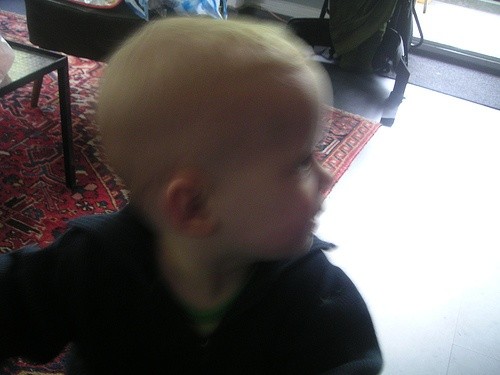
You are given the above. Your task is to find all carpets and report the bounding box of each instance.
[0,10,385,256]
[315,42,500,111]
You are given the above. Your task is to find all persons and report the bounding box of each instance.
[0,18,383,375]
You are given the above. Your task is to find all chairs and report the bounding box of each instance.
[26,0,157,107]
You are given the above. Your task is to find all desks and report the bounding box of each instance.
[0,38,75,186]
